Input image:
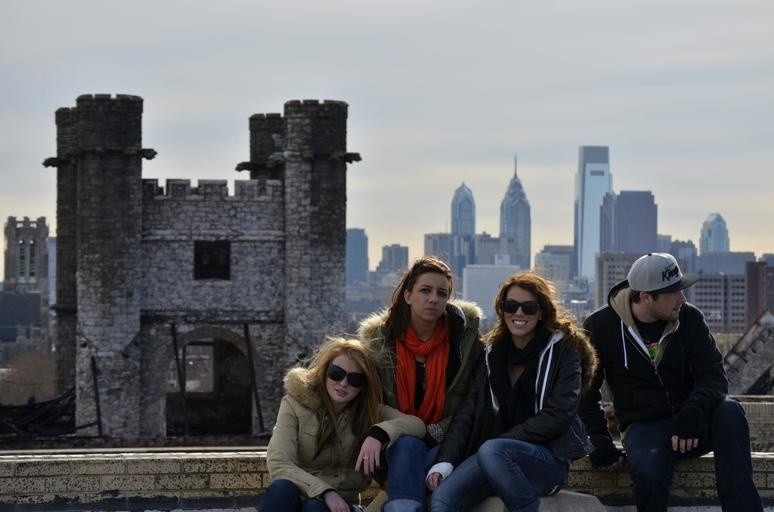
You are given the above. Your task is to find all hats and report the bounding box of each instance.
[626,253,700,294]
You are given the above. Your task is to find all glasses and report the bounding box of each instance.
[500,298,539,315]
[327,363,365,388]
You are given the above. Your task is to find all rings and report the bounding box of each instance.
[362,456,369,460]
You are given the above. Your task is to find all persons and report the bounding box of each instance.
[582,252,765,512]
[424,271,599,512]
[357,256,486,512]
[257,338,426,512]
